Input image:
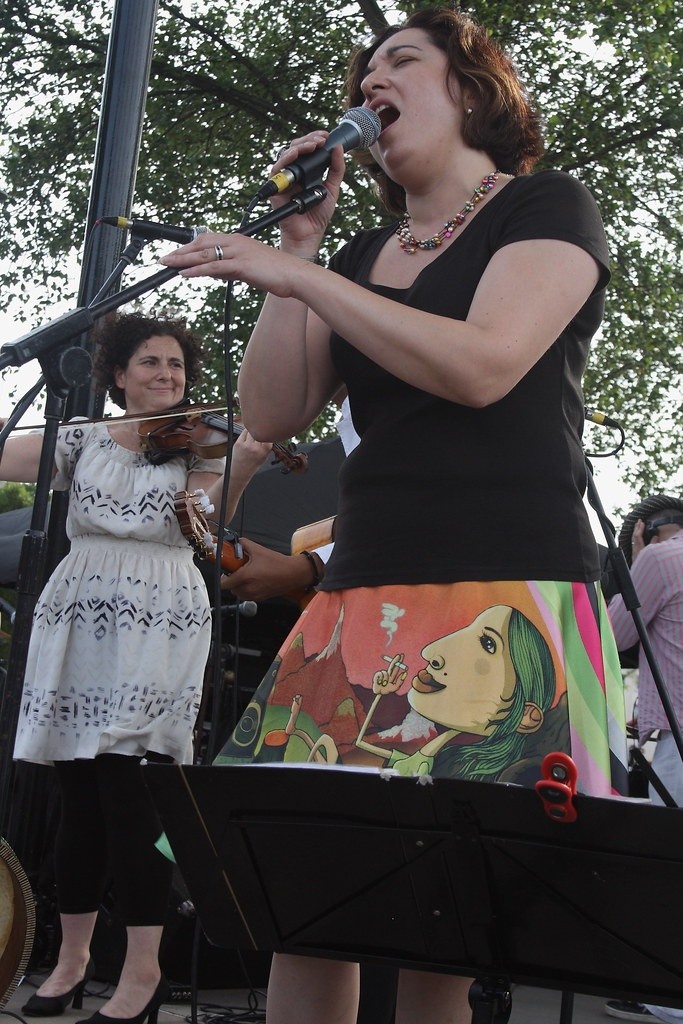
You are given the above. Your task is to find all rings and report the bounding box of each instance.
[214,246,224,260]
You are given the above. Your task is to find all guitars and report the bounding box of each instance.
[174,489,339,613]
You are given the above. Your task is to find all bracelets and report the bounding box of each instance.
[302,549,326,589]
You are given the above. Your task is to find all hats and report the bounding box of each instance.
[619,497,683,568]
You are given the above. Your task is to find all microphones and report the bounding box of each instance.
[210,600,258,617]
[253,107,382,201]
[96,215,214,245]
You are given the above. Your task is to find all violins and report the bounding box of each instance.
[136,399,309,475]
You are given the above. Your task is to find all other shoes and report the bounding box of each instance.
[603,1001,667,1024]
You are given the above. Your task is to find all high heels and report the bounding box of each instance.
[76,972,164,1023]
[21,978,88,1016]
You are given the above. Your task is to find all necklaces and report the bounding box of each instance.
[124,423,144,448]
[394,169,501,256]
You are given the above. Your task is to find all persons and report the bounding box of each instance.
[157,6,622,1024]
[600,494,683,1024]
[216,381,363,604]
[0,313,274,1024]
